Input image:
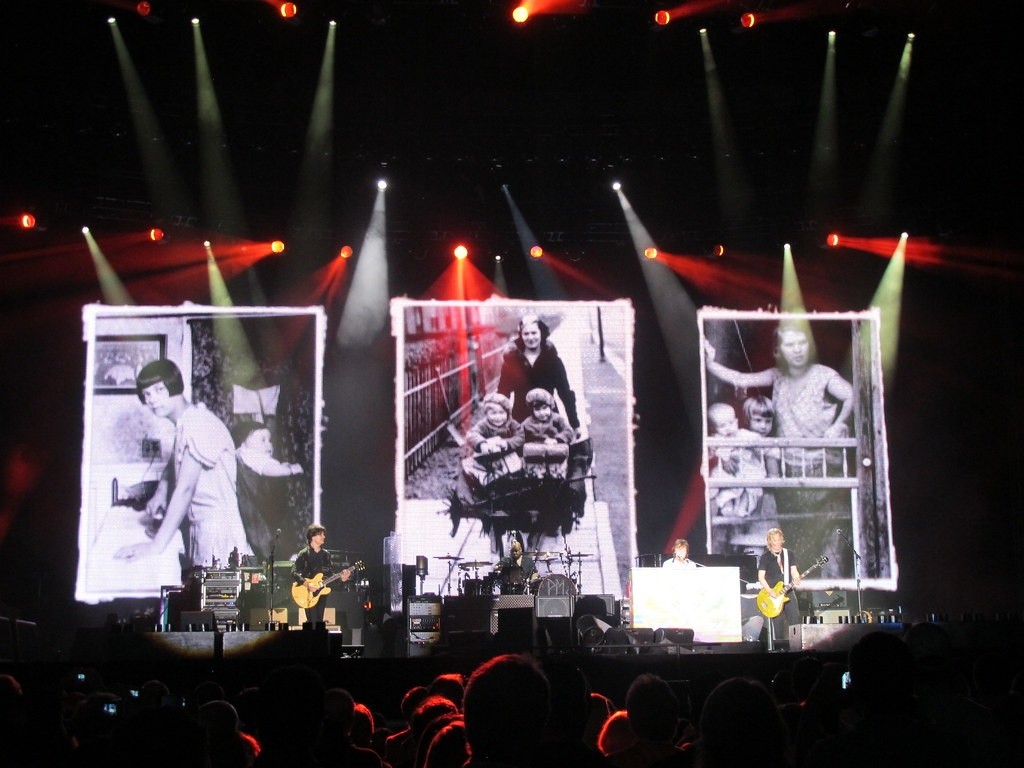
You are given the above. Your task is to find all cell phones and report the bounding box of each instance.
[842,671,852,690]
[130,690,140,697]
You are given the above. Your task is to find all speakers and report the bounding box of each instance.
[144,592,282,661]
[604,628,694,653]
[444,594,615,652]
[807,590,847,609]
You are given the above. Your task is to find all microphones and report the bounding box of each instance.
[511,530,516,537]
[837,529,843,534]
[277,529,281,536]
[678,552,685,560]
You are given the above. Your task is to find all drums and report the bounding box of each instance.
[501,566,523,587]
[462,579,485,596]
[527,574,577,598]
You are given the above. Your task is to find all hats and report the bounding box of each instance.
[483,392,512,414]
[526,389,555,409]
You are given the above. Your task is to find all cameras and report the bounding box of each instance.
[103,699,123,716]
[77,674,85,680]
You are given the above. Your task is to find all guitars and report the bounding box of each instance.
[757,555,829,619]
[292,559,366,609]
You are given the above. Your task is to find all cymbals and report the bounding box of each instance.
[432,556,464,560]
[459,562,493,567]
[525,552,560,562]
[565,554,593,557]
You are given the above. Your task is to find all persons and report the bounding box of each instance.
[495,541,535,580]
[757,527,801,650]
[662,539,702,568]
[0,610,1024,768]
[462,315,579,478]
[95,334,247,571]
[289,524,354,621]
[698,326,856,587]
